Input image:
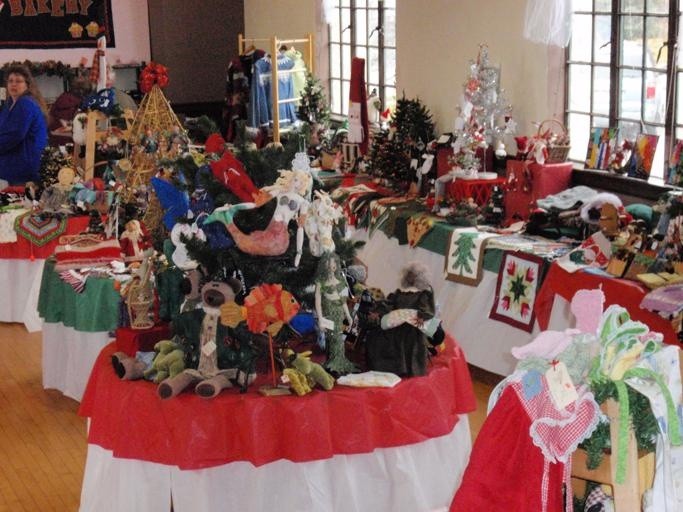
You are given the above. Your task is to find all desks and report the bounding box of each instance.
[83,323,469,512]
[42,235,127,402]
[329,169,681,388]
[1,191,110,331]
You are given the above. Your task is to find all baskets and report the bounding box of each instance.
[538,120,571,163]
[445,176,507,207]
[124,259,155,329]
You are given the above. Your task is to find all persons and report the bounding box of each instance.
[0,64,47,185]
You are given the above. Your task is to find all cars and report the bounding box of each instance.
[569,33,656,153]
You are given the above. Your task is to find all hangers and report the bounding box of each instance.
[227,39,306,73]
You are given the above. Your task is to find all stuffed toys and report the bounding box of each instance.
[107,132,445,402]
[106,132,445,402]
